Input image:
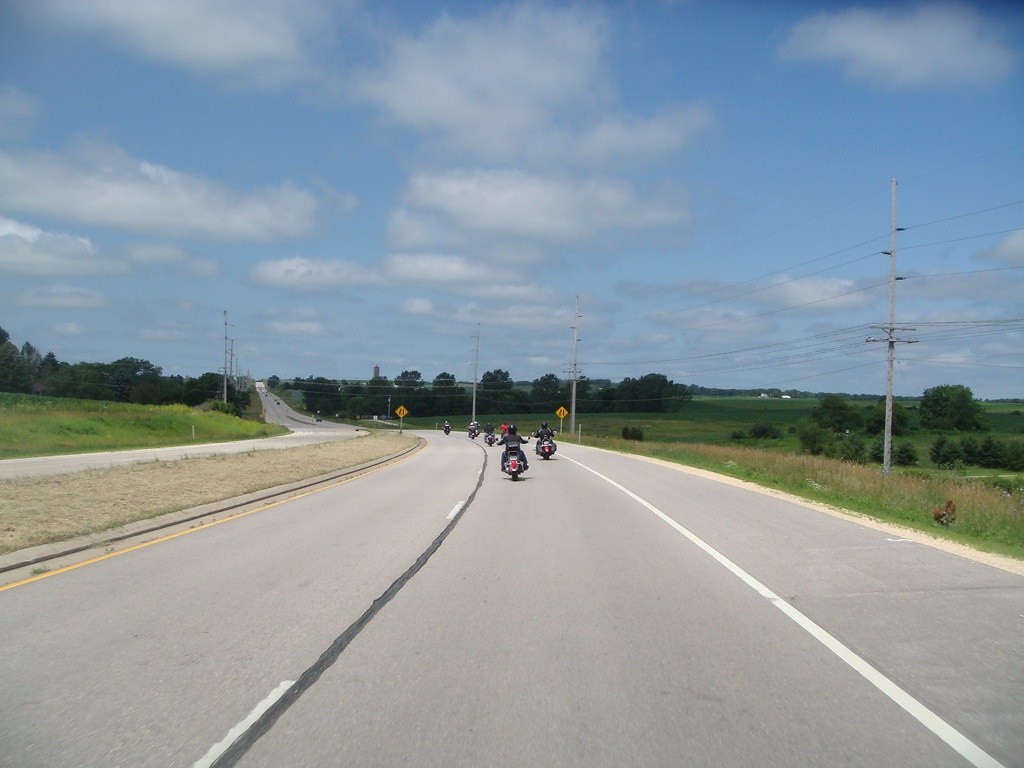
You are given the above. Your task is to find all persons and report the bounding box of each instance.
[468,421,479,437]
[534,422,556,455]
[443,421,451,433]
[484,423,495,443]
[500,423,509,438]
[497,424,529,471]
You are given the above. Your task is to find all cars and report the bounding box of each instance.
[315,418,321,421]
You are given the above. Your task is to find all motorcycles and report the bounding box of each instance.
[484,430,498,446]
[500,437,531,480]
[533,429,556,460]
[469,424,479,439]
[443,425,451,435]
[499,427,508,439]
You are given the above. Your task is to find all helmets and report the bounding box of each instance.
[508,424,517,435]
[487,423,491,426]
[470,423,474,426]
[474,421,477,424]
[542,422,548,429]
